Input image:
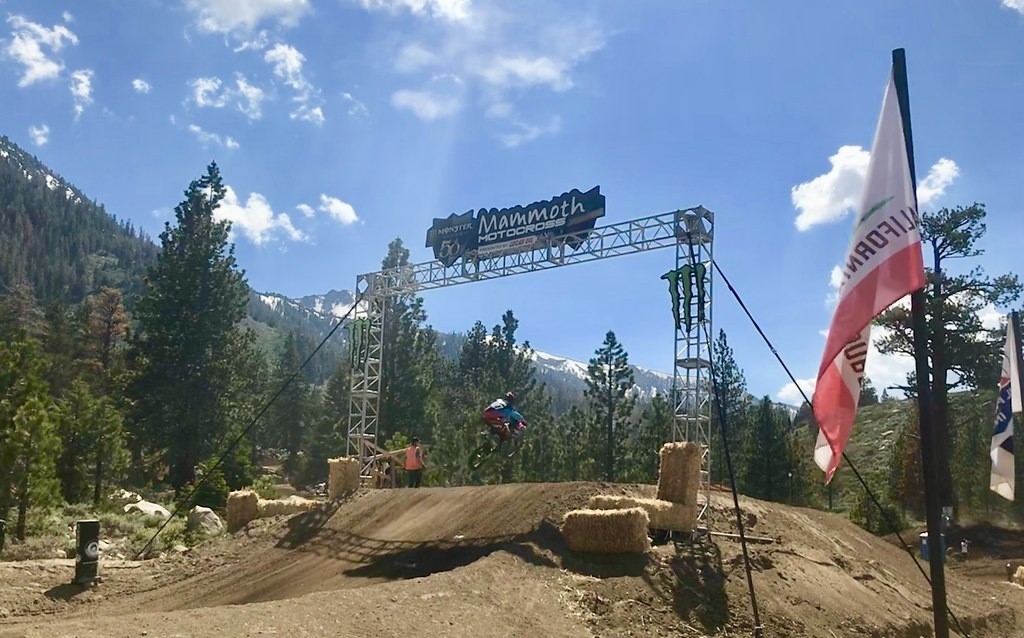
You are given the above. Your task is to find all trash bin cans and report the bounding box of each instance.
[75,520,100,582]
[0,520,6,552]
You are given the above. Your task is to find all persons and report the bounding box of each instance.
[480,391,528,458]
[405,437,430,488]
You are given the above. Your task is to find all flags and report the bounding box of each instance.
[989,316,1022,502]
[812,64,925,487]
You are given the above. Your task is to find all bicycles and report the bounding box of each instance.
[467,419,525,471]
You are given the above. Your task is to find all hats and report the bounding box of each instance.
[412,436,423,443]
[505,391,514,400]
